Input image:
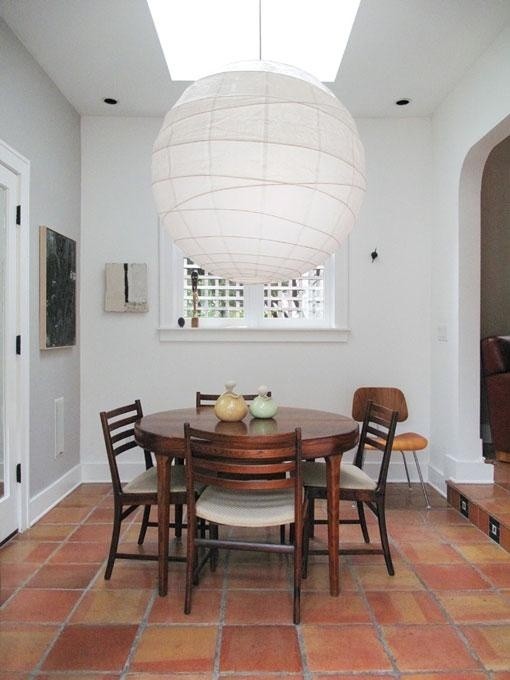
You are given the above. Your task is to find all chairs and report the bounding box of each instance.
[196,391,271,408]
[351,387,430,509]
[100,399,219,586]
[183,422,311,625]
[291,398,399,577]
[479,332,510,465]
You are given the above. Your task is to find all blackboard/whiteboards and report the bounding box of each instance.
[39,225,77,351]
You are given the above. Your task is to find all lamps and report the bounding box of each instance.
[149,0,367,284]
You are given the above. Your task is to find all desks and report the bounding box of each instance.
[133,405,360,596]
